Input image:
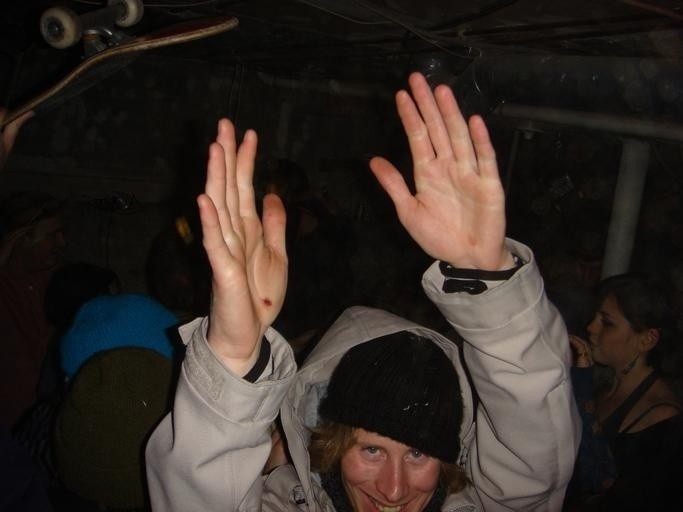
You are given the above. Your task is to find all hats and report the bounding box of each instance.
[317,331,463,465]
[52,292,184,383]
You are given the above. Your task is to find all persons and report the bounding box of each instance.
[0,224,212,511]
[256,157,354,371]
[562,272,681,510]
[0,107,71,437]
[144,69,582,511]
